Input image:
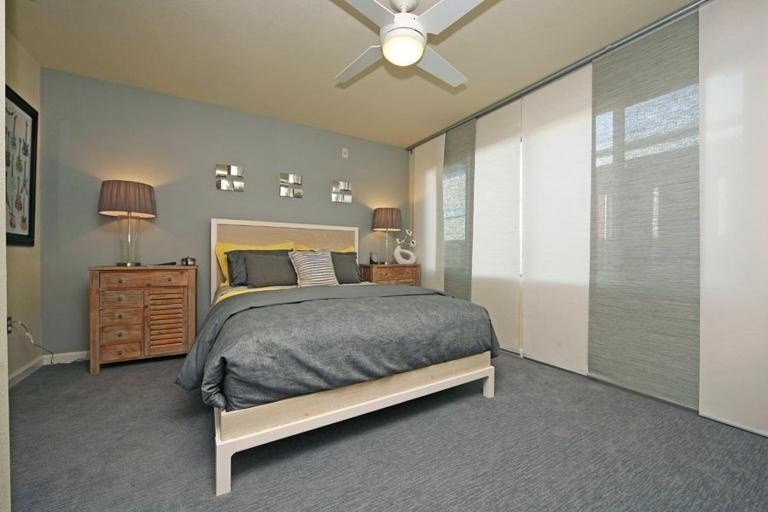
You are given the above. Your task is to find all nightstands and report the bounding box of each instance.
[359,263,420,287]
[89,265,196,374]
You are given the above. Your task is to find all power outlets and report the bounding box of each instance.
[7,317,13,333]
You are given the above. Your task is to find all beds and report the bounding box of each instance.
[177,219,499,496]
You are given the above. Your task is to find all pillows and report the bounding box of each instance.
[288,250,339,287]
[330,251,362,283]
[215,242,293,287]
[244,254,297,287]
[296,244,354,283]
[229,248,292,286]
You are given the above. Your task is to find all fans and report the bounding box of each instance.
[334,0,485,87]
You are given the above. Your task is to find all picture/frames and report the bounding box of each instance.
[5,83,39,247]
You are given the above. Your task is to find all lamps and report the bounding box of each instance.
[371,208,401,264]
[98,181,157,266]
[379,25,426,68]
